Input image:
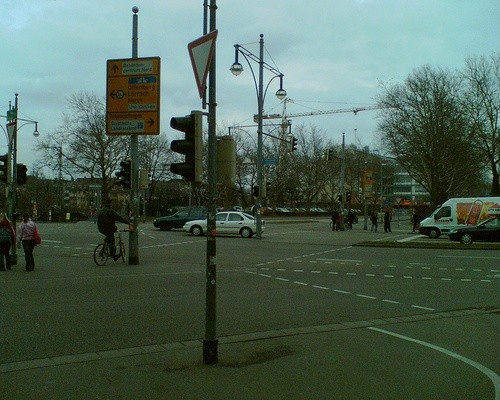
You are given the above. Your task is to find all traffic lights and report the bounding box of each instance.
[327,147,333,161]
[16,163,28,185]
[169,110,203,183]
[119,159,133,190]
[113,170,123,187]
[0,152,12,183]
[289,133,300,153]
[266,181,272,197]
[346,192,351,202]
[338,193,342,202]
[252,186,259,197]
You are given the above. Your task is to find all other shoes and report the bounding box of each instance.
[25,269,31,271]
[114,254,120,261]
[30,268,34,272]
[6,267,12,270]
[0,269,5,271]
[98,253,105,258]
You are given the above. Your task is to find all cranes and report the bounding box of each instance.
[254,98,409,177]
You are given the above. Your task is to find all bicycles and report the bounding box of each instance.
[93,223,127,267]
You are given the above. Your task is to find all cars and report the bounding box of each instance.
[447,213,500,246]
[153,207,218,231]
[182,211,266,238]
[216,206,361,216]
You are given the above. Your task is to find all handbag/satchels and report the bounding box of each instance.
[33,225,42,245]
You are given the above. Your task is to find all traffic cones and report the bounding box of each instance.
[229,30,287,238]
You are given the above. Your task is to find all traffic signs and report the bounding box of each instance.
[105,56,161,135]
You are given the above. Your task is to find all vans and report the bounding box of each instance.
[417,197,500,239]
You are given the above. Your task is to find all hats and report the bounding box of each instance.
[103,199,112,204]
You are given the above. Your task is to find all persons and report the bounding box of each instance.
[410,211,420,232]
[382,207,392,233]
[0,212,15,272]
[17,214,40,272]
[331,208,353,232]
[97,200,130,261]
[370,209,379,232]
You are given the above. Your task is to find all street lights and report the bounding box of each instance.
[9,119,39,265]
